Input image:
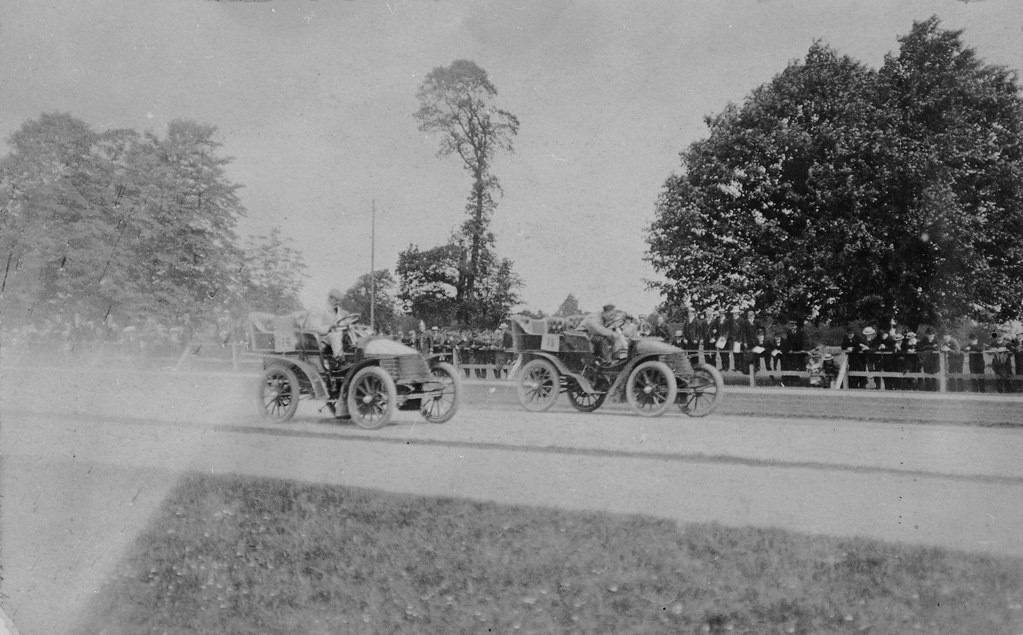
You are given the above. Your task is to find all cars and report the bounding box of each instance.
[256,311,461,429]
[509,312,724,420]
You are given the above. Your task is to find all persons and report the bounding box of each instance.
[577,302,673,368]
[917,326,939,392]
[740,308,775,382]
[549,319,562,335]
[963,334,987,393]
[380,318,513,381]
[302,287,349,363]
[685,305,744,372]
[870,329,906,391]
[938,329,964,393]
[900,331,922,389]
[771,318,811,388]
[843,325,882,391]
[671,330,689,358]
[989,329,1023,395]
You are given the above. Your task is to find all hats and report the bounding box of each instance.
[925,327,936,334]
[729,306,741,313]
[906,332,916,340]
[1014,328,1022,335]
[968,333,980,340]
[329,287,344,300]
[773,330,783,337]
[845,327,856,333]
[893,334,904,341]
[875,328,886,338]
[863,327,876,334]
